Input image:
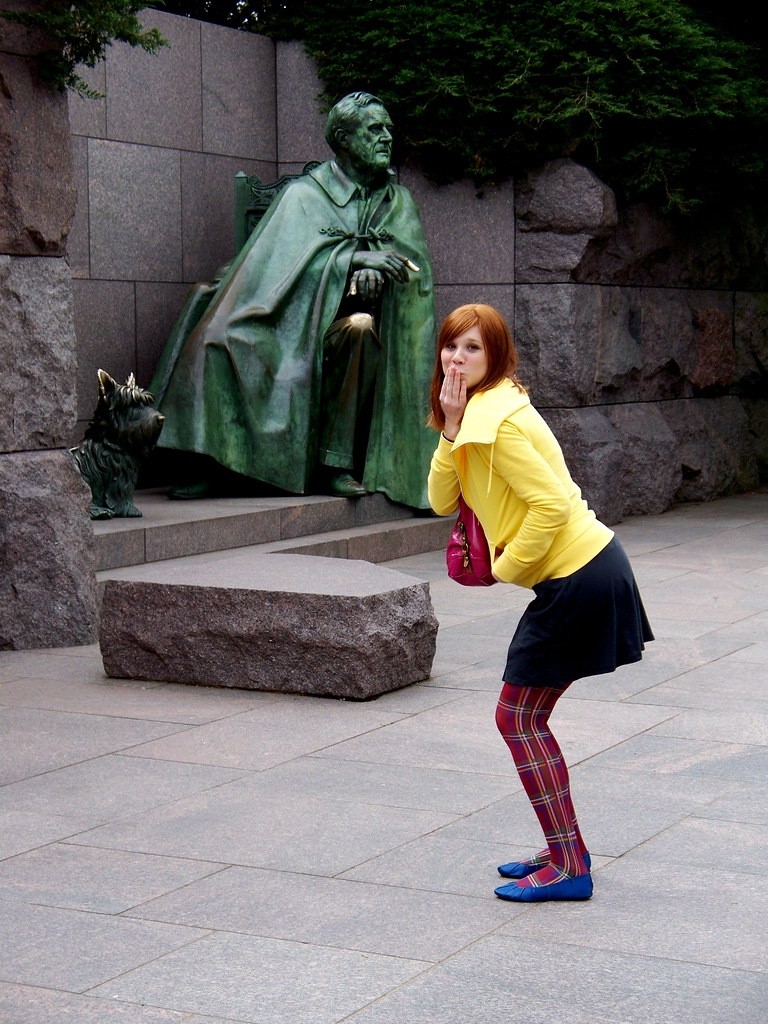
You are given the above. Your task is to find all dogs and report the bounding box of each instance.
[68,368,165,520]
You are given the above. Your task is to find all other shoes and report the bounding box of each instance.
[494,873,593,902]
[497,852,591,879]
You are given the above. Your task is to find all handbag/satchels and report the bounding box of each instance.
[446,494,499,586]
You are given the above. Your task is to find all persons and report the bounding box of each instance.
[142,89,442,510]
[425,302,655,902]
